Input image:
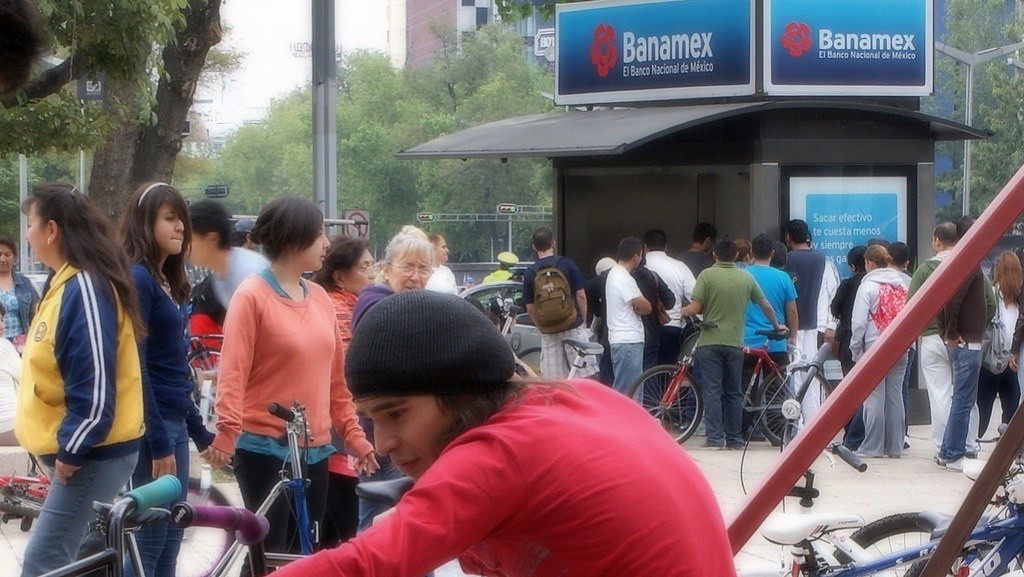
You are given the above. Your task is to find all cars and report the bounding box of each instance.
[459,280,542,365]
[25,274,47,296]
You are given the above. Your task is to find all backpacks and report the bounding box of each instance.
[527,255,578,334]
[868,282,907,335]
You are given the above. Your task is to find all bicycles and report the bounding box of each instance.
[500,299,604,381]
[0,470,50,532]
[624,314,832,446]
[186,334,225,421]
[758,400,1024,577]
[37,400,415,576]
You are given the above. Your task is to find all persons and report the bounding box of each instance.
[0,197,1024,577]
[198,194,382,577]
[266,287,735,577]
[115,179,216,577]
[13,182,147,577]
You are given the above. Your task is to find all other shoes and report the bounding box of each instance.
[698,440,749,450]
[937,455,972,472]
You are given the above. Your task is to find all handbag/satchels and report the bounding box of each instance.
[657,297,672,326]
[982,318,1012,374]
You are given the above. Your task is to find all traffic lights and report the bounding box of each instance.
[418,213,436,223]
[498,203,518,215]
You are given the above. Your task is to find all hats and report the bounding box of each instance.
[498,251,519,266]
[343,289,515,394]
